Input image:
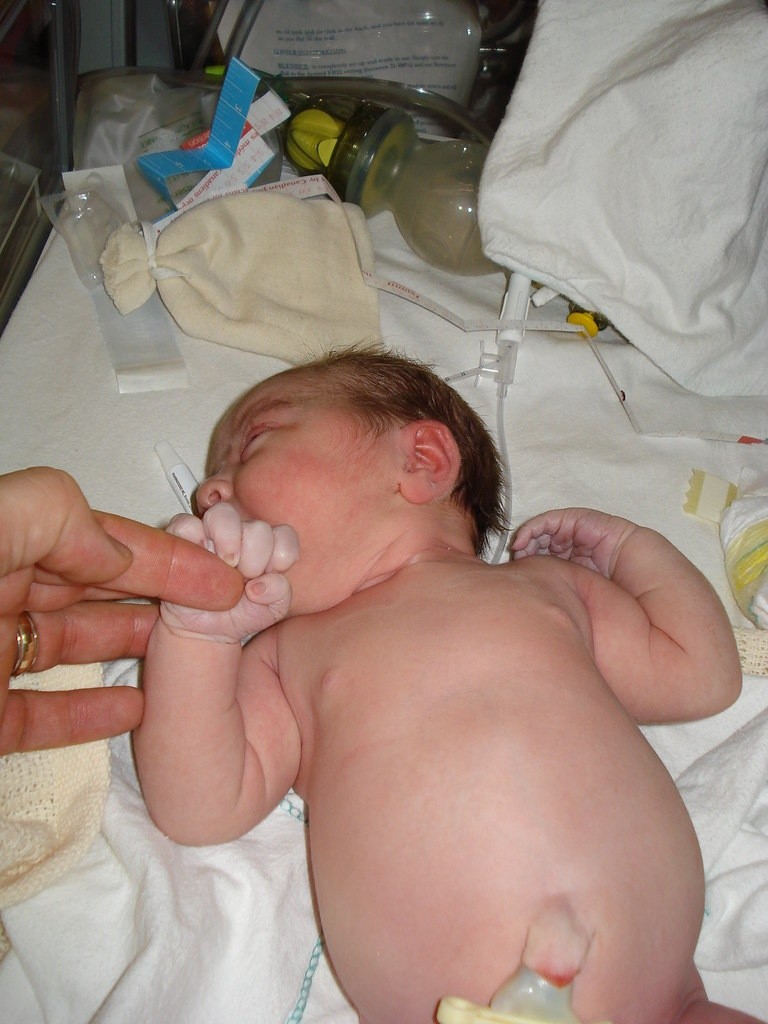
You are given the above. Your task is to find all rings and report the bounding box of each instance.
[12,611,38,678]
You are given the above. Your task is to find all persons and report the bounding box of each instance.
[0,467,245,759]
[130,340,767,1024]
